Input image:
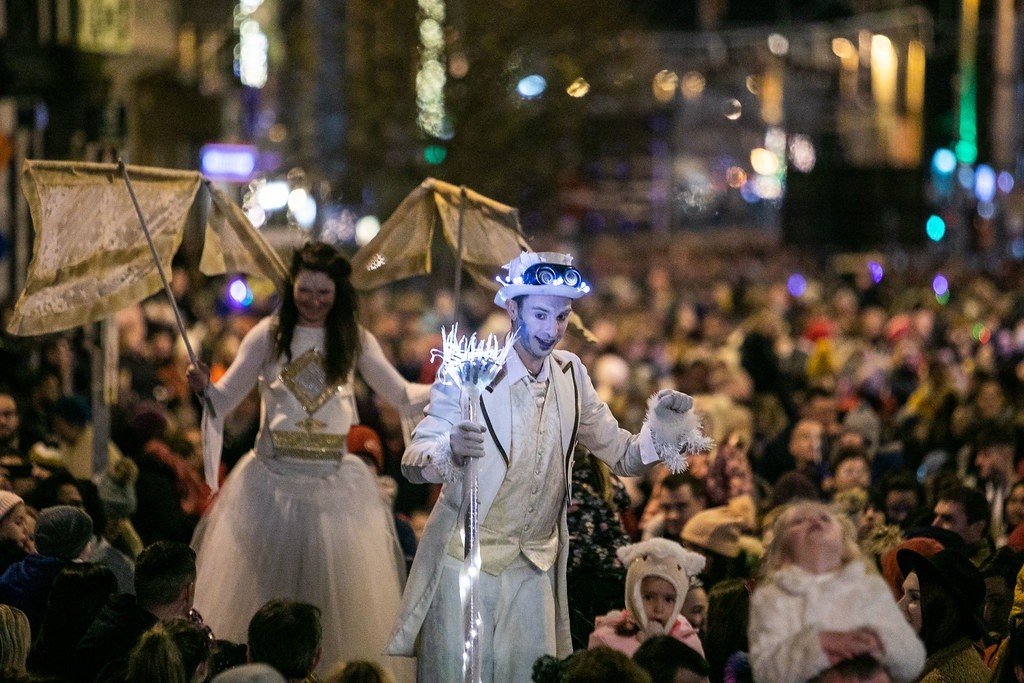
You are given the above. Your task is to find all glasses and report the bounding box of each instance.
[186,609,218,646]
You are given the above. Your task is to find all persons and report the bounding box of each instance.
[0,240,1024,683]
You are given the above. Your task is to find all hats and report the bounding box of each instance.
[0,490,25,521]
[34,505,93,559]
[680,505,746,558]
[493,251,590,310]
[896,548,986,641]
[616,538,706,638]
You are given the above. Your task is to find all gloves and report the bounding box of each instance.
[653,388,694,421]
[450,420,487,467]
